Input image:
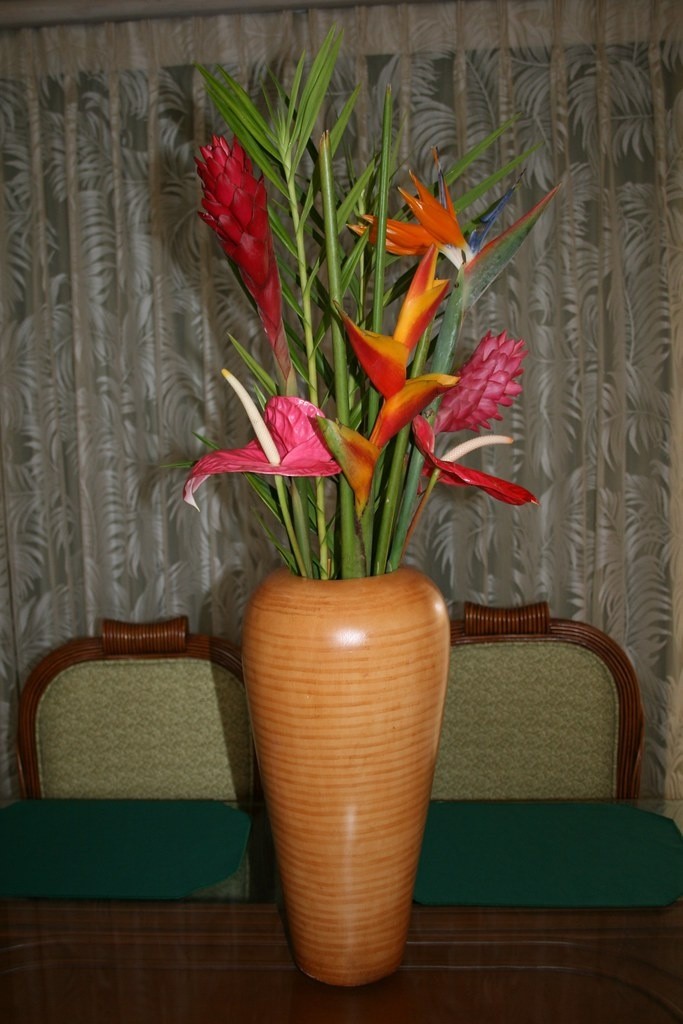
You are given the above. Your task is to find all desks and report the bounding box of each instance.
[0,898,683,1024]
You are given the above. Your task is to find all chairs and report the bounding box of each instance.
[0,614,276,903]
[410,602,682,910]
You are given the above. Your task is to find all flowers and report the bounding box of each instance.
[158,19,564,582]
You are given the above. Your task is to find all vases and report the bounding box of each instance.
[241,569,453,988]
